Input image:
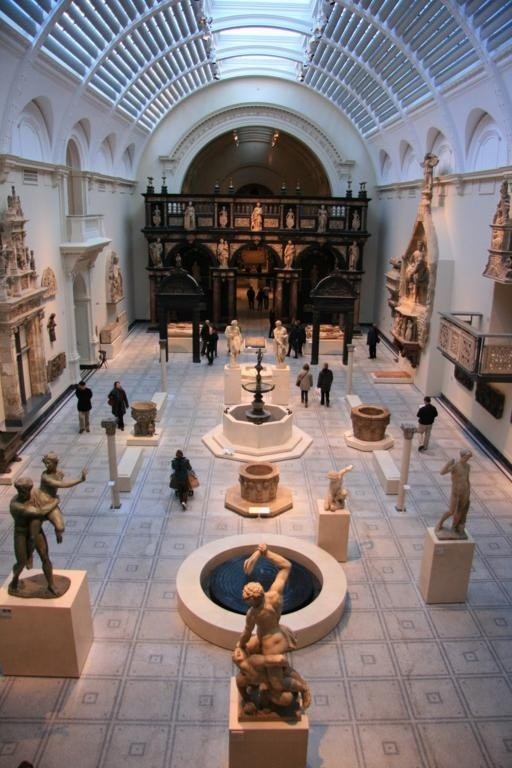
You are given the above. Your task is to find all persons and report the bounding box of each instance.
[24,450,89,570]
[230,646,303,725]
[216,237,230,270]
[404,239,429,301]
[153,204,162,227]
[184,200,196,231]
[317,204,328,233]
[351,209,360,231]
[74,381,93,435]
[285,207,295,229]
[224,318,243,368]
[106,380,131,433]
[272,319,289,369]
[414,395,439,452]
[232,543,312,710]
[322,464,354,513]
[435,447,474,533]
[256,287,265,312]
[205,327,219,366]
[294,363,314,408]
[268,307,277,339]
[5,476,62,597]
[169,449,193,511]
[251,201,264,231]
[212,328,219,358]
[112,257,123,298]
[149,237,163,268]
[283,240,296,270]
[246,286,256,313]
[317,362,334,408]
[200,318,213,356]
[218,206,228,228]
[348,241,360,272]
[365,323,380,360]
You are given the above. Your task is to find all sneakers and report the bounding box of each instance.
[417,445,424,450]
[179,500,188,512]
[118,425,125,432]
[79,428,90,434]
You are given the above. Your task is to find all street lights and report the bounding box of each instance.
[395,421,416,512]
[158,338,170,399]
[346,343,357,397]
[100,416,122,508]
